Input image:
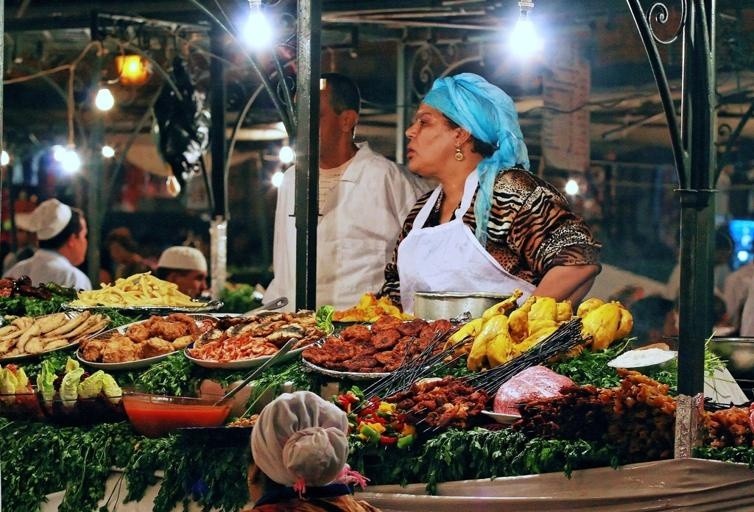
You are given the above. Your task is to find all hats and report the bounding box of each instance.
[158,246,207,272]
[29,198,72,241]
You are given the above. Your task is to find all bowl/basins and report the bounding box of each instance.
[123,390,236,434]
[34,387,140,426]
[663,331,753,377]
[0,383,47,422]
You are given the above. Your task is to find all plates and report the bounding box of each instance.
[20,306,112,361]
[76,313,220,368]
[301,319,451,383]
[57,299,225,313]
[478,408,528,427]
[182,307,335,367]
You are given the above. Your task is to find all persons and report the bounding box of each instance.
[0,199,231,296]
[371,72,603,322]
[625,231,754,348]
[240,388,382,511]
[259,72,433,310]
